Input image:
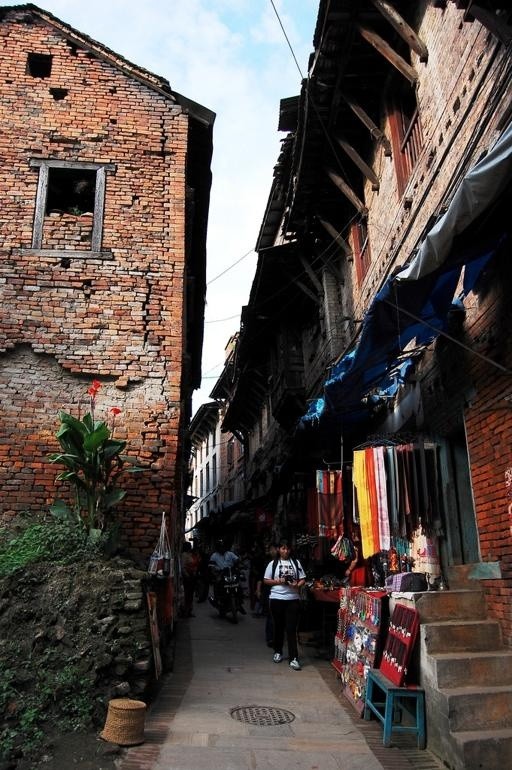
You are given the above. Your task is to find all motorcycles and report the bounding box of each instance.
[208,565,248,625]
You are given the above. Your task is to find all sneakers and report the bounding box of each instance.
[274,652,281,663]
[291,658,300,669]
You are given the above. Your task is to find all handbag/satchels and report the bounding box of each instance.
[391,572,410,592]
[401,573,428,591]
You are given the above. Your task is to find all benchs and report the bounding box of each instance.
[364,669,426,749]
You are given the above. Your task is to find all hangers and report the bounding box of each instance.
[353,430,422,451]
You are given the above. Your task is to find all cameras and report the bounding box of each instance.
[284,575,293,585]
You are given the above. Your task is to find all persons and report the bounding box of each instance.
[180,533,369,619]
[264,540,307,672]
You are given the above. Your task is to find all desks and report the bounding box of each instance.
[303,582,345,659]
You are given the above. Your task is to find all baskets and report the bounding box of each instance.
[101,699,147,745]
